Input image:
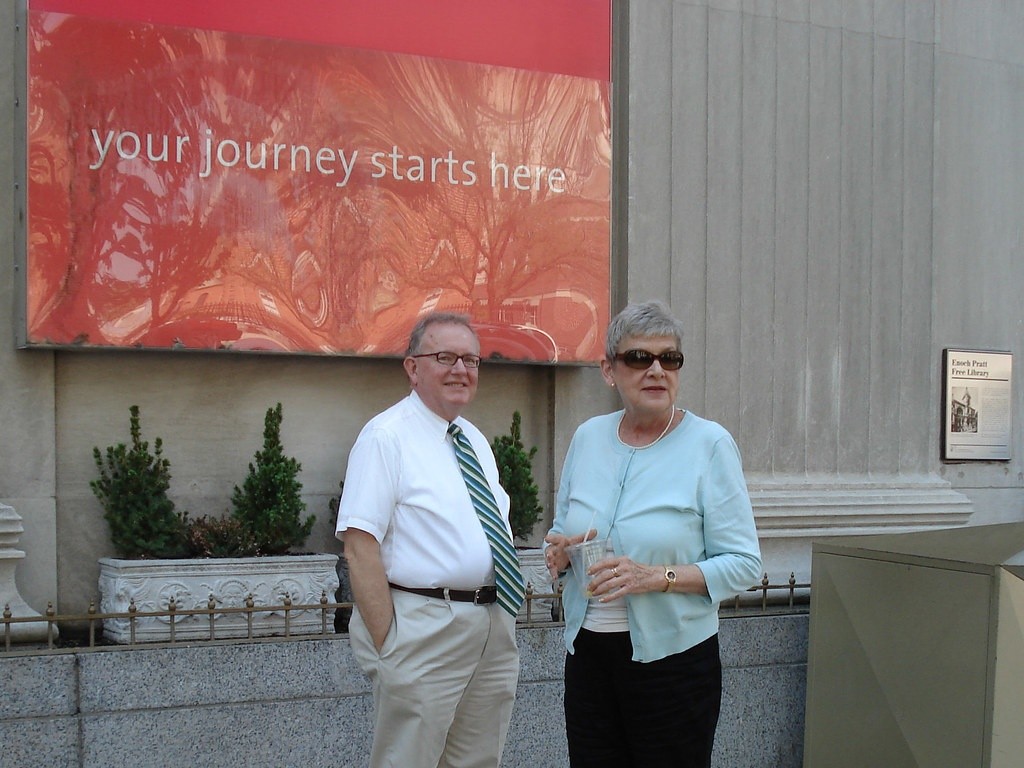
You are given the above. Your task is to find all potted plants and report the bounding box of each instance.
[89,403,340,646]
[329,412,553,623]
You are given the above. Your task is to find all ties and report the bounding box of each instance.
[446,423,526,619]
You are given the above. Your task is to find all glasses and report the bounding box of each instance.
[614,348,684,371]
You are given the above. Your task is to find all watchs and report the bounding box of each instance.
[663,565,677,594]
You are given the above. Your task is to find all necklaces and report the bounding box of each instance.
[617,405,675,449]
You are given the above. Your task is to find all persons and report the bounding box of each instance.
[541,300,765,768]
[335,312,525,768]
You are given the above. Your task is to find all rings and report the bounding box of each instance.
[612,568,620,576]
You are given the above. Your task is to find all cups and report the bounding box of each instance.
[564,539,611,599]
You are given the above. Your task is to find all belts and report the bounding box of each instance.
[388,581,497,604]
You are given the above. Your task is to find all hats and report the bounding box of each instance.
[412,351,482,368]
